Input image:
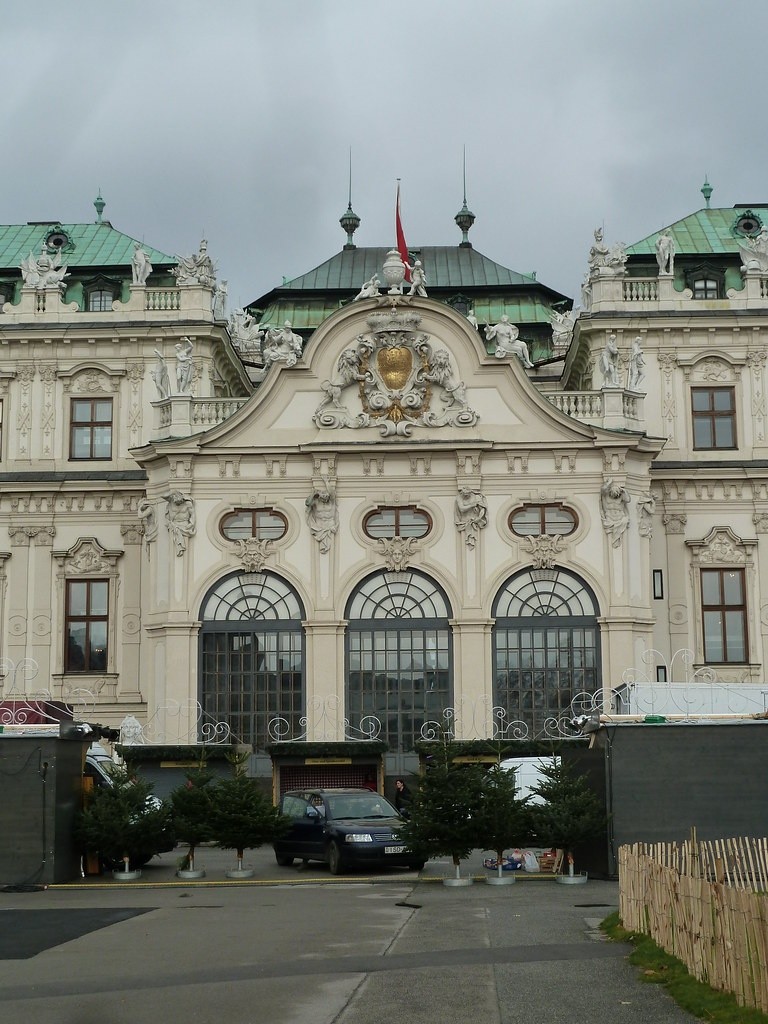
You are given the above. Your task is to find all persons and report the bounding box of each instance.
[214,278,228,321]
[636,488,658,538]
[161,489,196,557]
[600,333,619,388]
[118,714,143,745]
[587,228,609,269]
[452,486,488,549]
[304,475,338,555]
[481,314,535,369]
[465,309,479,331]
[602,478,628,549]
[354,275,384,301]
[173,337,194,393]
[654,227,676,275]
[404,258,429,298]
[151,348,171,400]
[261,319,302,375]
[632,336,645,391]
[129,241,151,286]
[134,497,160,545]
[395,779,415,825]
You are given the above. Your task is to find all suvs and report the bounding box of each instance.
[270,785,430,876]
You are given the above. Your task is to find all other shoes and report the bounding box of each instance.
[297,864,309,870]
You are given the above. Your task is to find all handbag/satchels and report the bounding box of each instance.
[523,851,541,872]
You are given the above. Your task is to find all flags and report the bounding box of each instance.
[395,200,414,285]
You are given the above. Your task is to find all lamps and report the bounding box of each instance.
[569,714,580,731]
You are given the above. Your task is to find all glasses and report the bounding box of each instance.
[314,799,320,801]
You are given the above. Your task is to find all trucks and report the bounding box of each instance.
[83,740,175,870]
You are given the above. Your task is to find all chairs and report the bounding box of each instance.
[350,802,369,816]
[334,803,349,817]
